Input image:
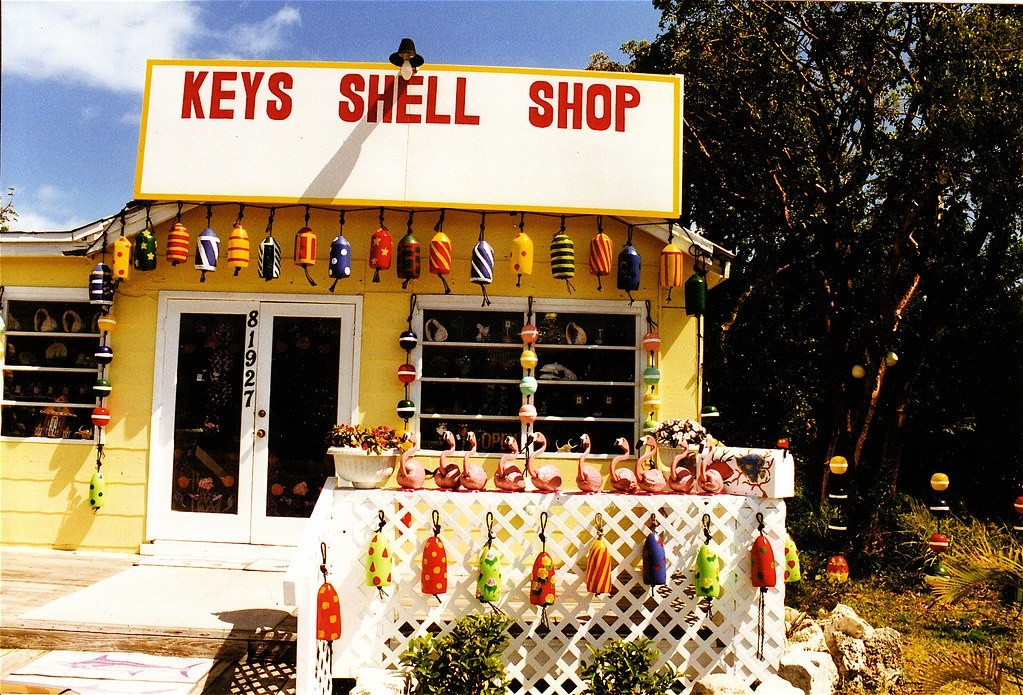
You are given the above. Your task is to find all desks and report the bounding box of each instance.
[333,490,789,695]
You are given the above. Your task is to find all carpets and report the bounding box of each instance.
[21,564,298,633]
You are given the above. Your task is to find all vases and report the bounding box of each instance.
[326,448,401,489]
[658,445,703,467]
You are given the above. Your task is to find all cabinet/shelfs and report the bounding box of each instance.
[0,330,104,443]
[421,341,634,427]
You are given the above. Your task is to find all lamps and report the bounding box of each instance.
[388,38,424,80]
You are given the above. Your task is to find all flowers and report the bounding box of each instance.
[325,423,416,454]
[650,419,714,449]
